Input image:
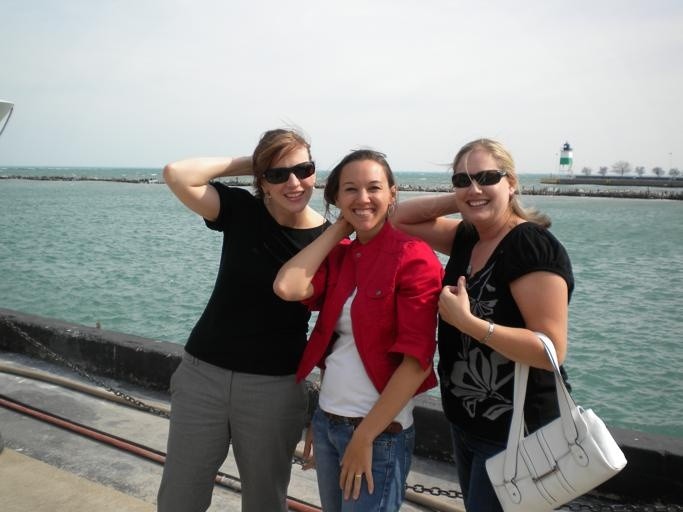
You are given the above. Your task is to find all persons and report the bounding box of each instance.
[273,149,445,512]
[391,138,575,512]
[155,129,350,512]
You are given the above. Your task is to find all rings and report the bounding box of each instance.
[355,474,362,477]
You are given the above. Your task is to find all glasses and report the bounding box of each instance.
[451,170,507,188]
[260,162,316,184]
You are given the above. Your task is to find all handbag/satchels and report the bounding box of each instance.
[486,406,628,512]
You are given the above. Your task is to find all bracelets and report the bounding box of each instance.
[478,320,494,345]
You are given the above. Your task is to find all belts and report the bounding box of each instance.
[324,411,403,434]
